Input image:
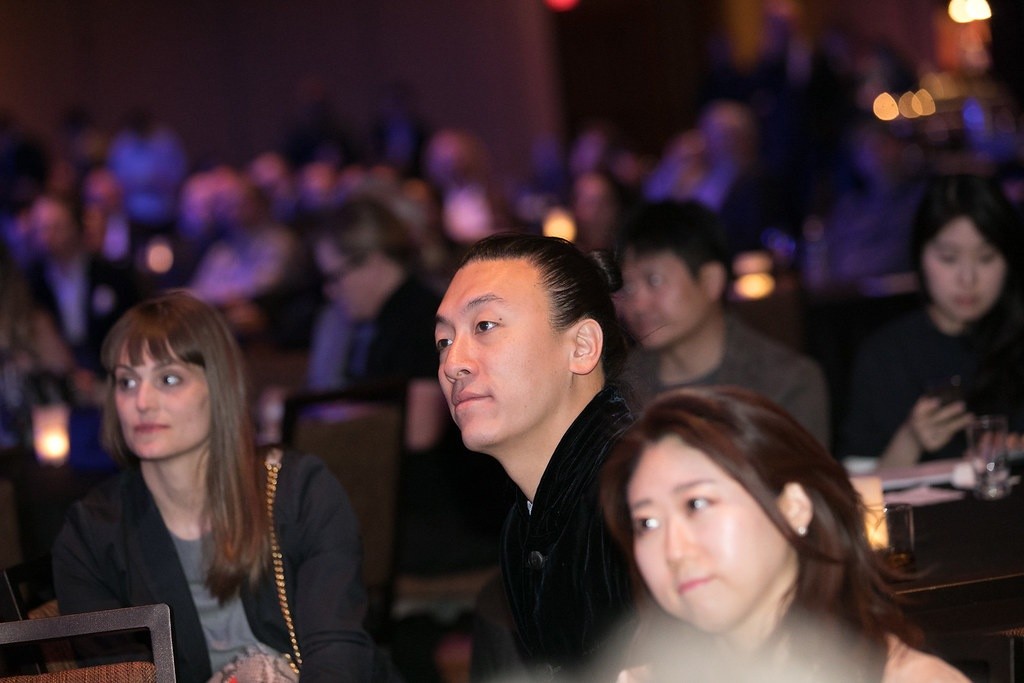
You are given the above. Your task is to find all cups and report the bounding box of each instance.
[966,413,1012,503]
[864,501,920,582]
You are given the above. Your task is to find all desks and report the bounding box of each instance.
[865,458,1024,683]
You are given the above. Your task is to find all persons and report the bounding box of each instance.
[0,0,1024,683]
